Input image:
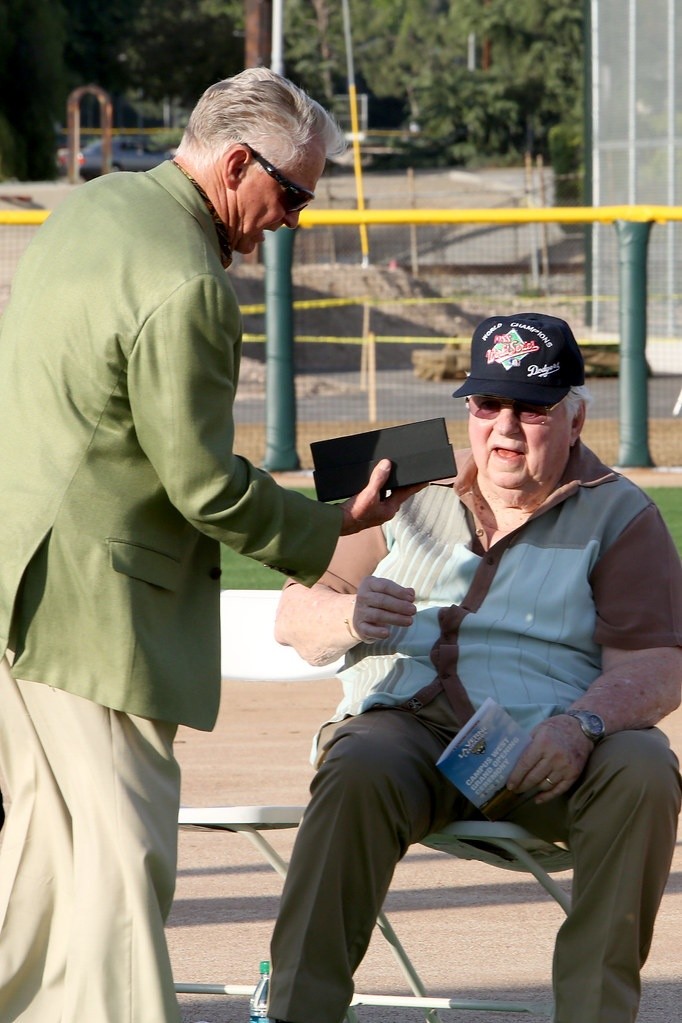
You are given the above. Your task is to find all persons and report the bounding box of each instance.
[0,66,429,1023]
[267,312,682,1023]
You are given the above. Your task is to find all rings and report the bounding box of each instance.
[545,777,555,786]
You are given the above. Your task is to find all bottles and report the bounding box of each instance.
[250,960,271,1023]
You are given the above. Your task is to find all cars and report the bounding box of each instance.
[57,134,171,182]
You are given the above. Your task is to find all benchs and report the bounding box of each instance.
[170,805,574,1023]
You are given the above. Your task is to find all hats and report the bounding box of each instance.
[452,312,586,402]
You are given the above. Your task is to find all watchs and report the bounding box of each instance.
[567,709,605,743]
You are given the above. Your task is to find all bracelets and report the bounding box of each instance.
[343,598,363,643]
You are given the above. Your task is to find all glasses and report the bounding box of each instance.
[242,140,314,215]
[466,394,569,425]
